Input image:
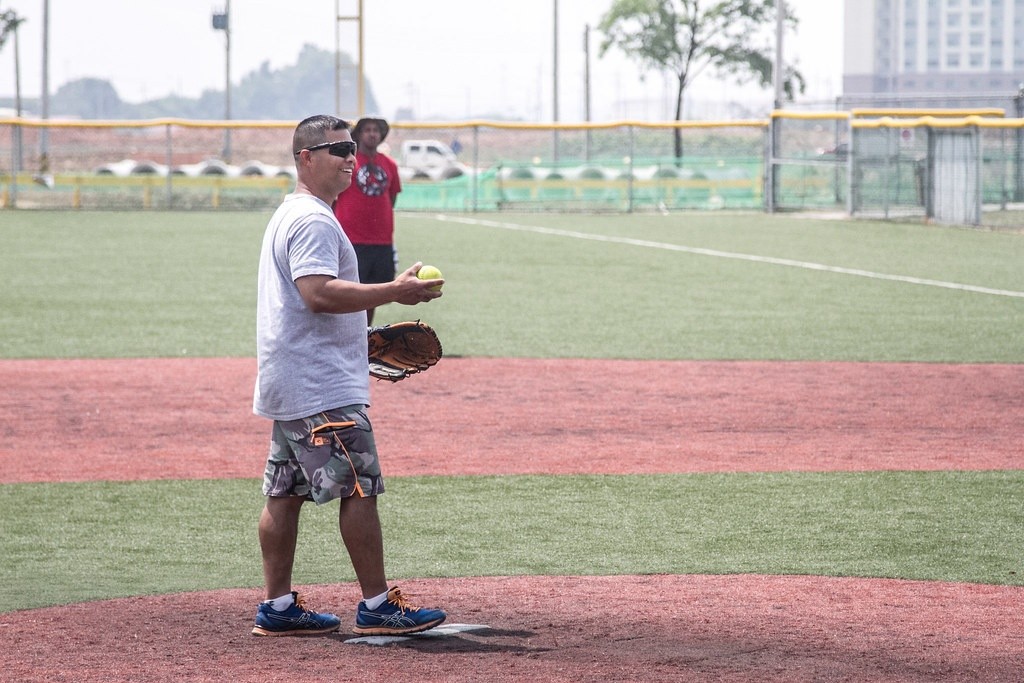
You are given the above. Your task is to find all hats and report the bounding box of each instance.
[350,118,389,144]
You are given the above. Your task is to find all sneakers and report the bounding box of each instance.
[353,586,446,635]
[252,591,340,637]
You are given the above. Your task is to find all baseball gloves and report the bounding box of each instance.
[366,320,442,383]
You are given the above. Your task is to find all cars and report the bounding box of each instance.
[820,143,848,156]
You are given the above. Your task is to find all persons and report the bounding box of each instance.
[333,117,401,327]
[251,115,445,635]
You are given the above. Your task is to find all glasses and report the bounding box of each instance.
[296,140,357,158]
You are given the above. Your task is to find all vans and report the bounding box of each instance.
[400,140,458,167]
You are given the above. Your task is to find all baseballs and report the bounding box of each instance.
[416,264,443,291]
